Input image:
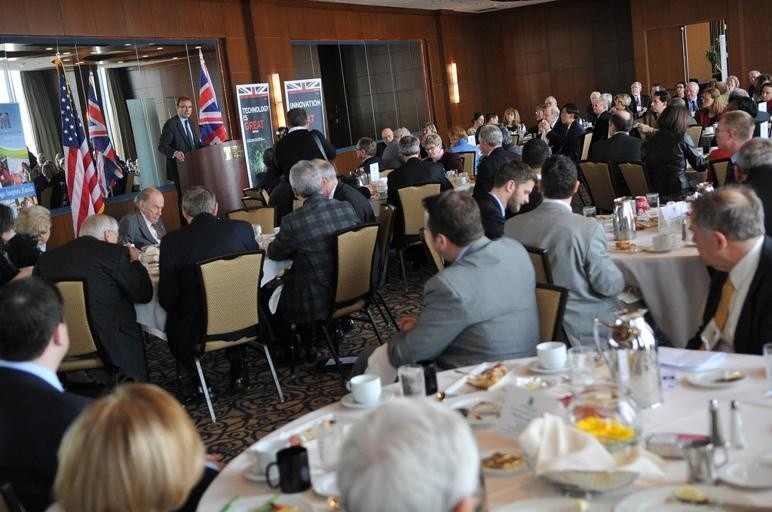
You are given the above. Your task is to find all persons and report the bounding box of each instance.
[32,214,153,387]
[46,383,206,512]
[158,96,211,229]
[257,69,771,312]
[118,186,167,251]
[155,185,259,402]
[0,276,226,512]
[0,203,35,281]
[28,145,128,211]
[501,154,654,349]
[338,398,484,512]
[256,160,363,367]
[6,203,52,269]
[0,154,31,186]
[349,189,540,383]
[682,184,772,359]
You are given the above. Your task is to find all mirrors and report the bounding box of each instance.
[0,35,234,225]
[288,38,433,155]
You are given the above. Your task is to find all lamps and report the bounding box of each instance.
[446,62,460,105]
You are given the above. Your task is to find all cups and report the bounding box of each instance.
[347,166,389,200]
[445,170,470,187]
[579,180,718,251]
[507,122,536,141]
[249,222,280,250]
[576,118,593,130]
[234,339,772,512]
[692,145,719,157]
[126,241,161,274]
[704,120,721,134]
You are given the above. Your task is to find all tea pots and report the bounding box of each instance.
[593,304,664,411]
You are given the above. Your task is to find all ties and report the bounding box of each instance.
[694,275,740,355]
[183,119,196,153]
[150,222,164,243]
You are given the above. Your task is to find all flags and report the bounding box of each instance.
[198,54,230,146]
[56,58,106,239]
[87,75,125,198]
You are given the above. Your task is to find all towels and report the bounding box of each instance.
[518,411,640,492]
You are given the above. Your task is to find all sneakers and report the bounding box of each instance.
[326,317,359,343]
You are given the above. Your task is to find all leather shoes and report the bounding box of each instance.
[174,382,219,413]
[226,366,249,395]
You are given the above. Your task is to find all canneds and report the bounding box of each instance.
[635,195,648,213]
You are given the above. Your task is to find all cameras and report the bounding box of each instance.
[276,126,289,140]
